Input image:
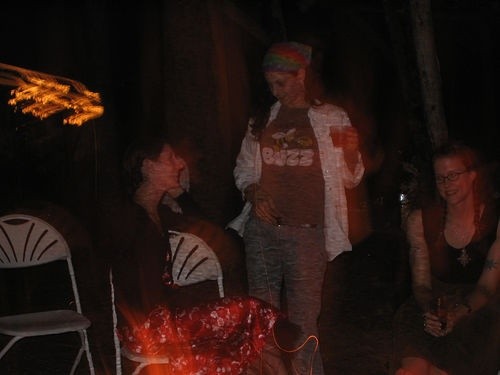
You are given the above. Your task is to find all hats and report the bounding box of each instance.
[261,41,312,74]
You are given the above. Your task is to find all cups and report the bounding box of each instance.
[430,296,449,330]
[330,125,348,146]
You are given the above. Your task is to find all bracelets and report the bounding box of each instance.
[460,301,472,316]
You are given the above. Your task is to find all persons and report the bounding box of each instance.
[394,144,500,375]
[112,41,384,375]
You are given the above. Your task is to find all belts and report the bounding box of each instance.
[275,217,320,228]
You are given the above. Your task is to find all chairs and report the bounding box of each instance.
[0,214,96,375]
[110,230,224,375]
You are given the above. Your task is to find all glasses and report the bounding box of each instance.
[434,171,470,184]
